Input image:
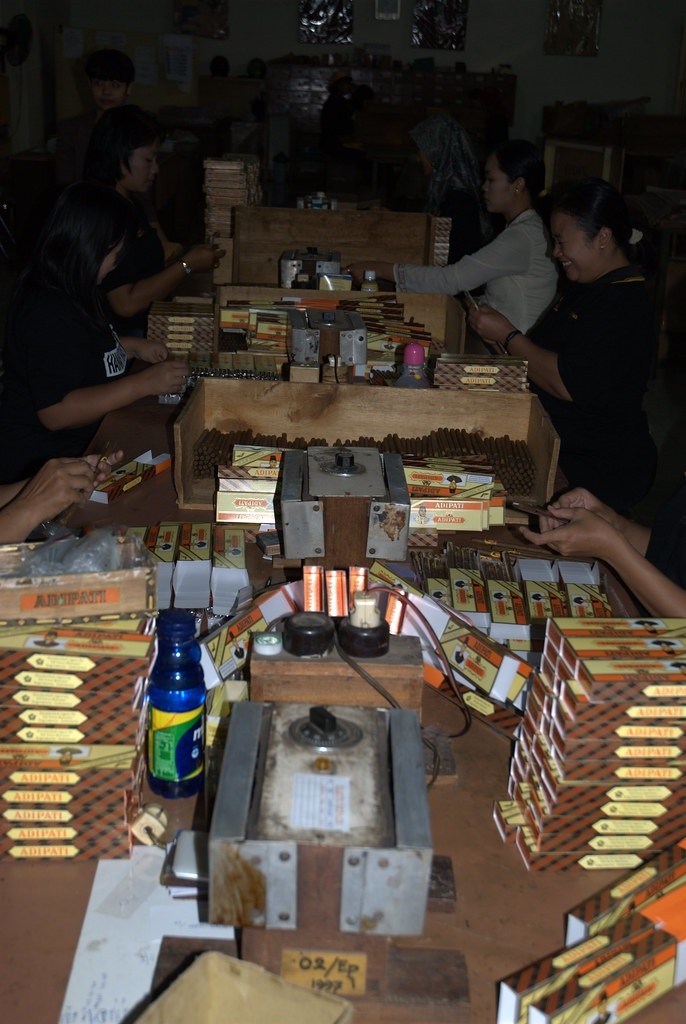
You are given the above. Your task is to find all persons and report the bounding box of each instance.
[320,71,397,205]
[341,140,560,354]
[58,48,181,260]
[519,478,686,617]
[81,103,226,335]
[468,177,659,512]
[0,183,192,484]
[408,113,497,299]
[0,455,111,546]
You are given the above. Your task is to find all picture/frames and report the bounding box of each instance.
[375,0,401,21]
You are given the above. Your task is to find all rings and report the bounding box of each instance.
[346,265,350,273]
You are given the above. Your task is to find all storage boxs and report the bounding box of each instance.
[0,537,160,860]
[105,522,263,616]
[87,449,171,504]
[319,273,352,290]
[367,323,532,391]
[204,153,264,238]
[405,462,509,533]
[200,447,613,827]
[492,615,686,1024]
[148,297,309,404]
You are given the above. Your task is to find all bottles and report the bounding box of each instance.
[147,609,207,799]
[362,271,378,292]
[396,343,431,389]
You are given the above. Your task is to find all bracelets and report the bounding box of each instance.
[504,330,521,348]
[181,259,190,278]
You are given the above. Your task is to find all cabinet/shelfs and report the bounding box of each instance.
[264,62,517,211]
